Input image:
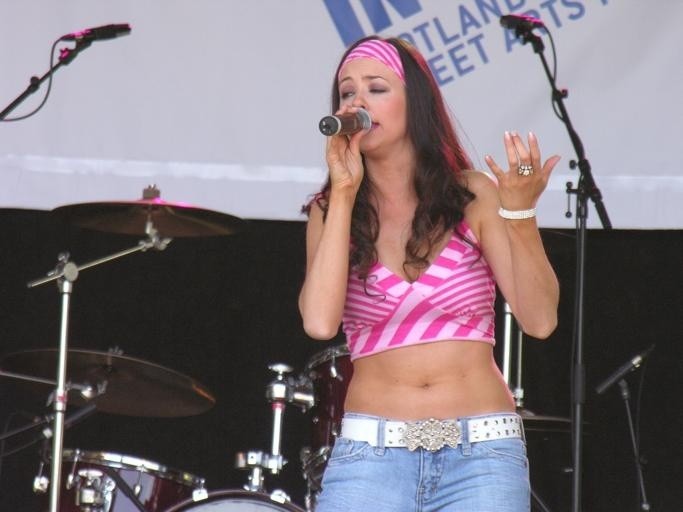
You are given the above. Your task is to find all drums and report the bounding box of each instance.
[300,344,354,493]
[33,444,206,512]
[163,488,305,512]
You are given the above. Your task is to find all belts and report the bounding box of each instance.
[338,414,519,448]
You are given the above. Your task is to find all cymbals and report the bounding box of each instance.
[512,410,594,437]
[51,199,247,238]
[0,346,216,417]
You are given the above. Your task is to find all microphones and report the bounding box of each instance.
[500,15,542,30]
[319,108,372,137]
[64,23,132,42]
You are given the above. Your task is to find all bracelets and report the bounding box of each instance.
[497,206,537,220]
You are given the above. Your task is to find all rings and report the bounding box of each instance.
[516,164,533,178]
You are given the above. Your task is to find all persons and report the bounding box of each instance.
[297,34,560,512]
